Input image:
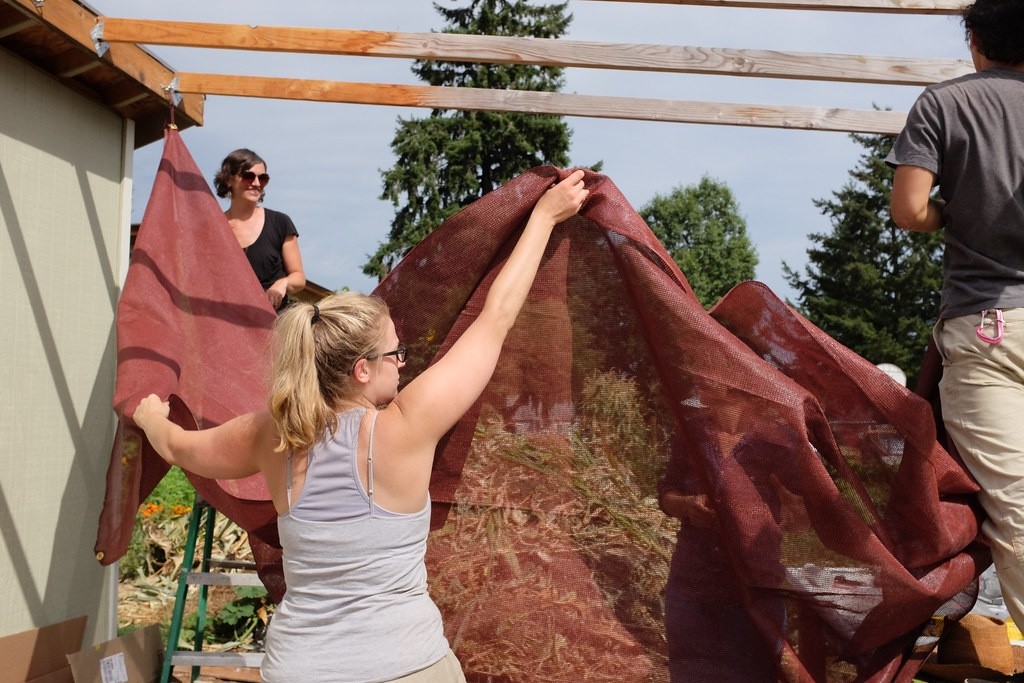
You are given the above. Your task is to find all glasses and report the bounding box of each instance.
[233,170,270,187]
[346,344,410,376]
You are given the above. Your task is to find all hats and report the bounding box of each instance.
[915,607,1024,682]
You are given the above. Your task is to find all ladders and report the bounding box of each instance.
[160,489,265,683]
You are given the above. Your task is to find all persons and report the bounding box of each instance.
[213,148,307,314]
[882,0,1023,638]
[133,170,590,683]
